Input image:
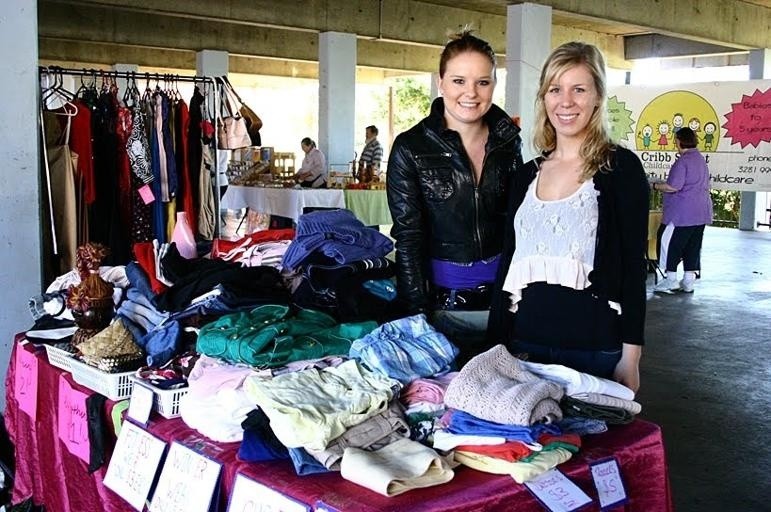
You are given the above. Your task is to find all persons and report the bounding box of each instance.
[293,136,328,188]
[485,38,654,399]
[384,21,526,351]
[357,124,383,179]
[643,125,713,295]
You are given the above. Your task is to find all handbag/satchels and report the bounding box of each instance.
[213,76,263,151]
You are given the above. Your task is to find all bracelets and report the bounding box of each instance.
[653,182,659,190]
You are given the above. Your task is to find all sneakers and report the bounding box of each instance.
[652,278,681,293]
[679,278,693,293]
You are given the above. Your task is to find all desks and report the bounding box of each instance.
[228,178,394,235]
[13,331,664,509]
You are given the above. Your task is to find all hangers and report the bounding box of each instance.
[36,64,203,118]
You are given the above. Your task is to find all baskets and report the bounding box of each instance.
[43,344,75,373]
[128,372,190,421]
[69,358,138,403]
